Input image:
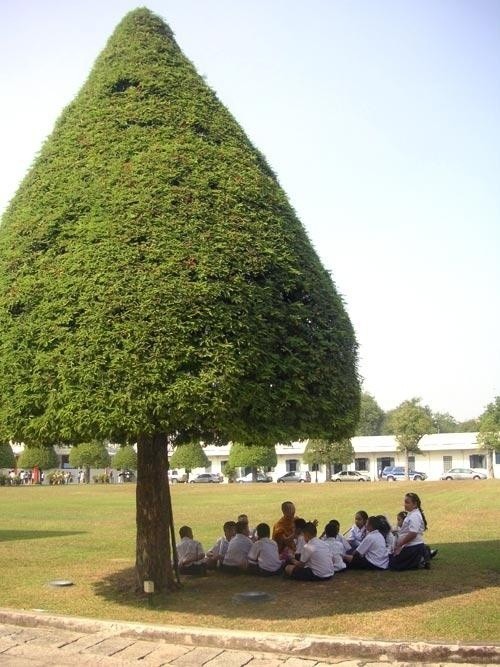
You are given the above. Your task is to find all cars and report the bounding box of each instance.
[236,471,272,482]
[441,467,487,480]
[330,470,371,482]
[189,472,224,483]
[277,470,311,482]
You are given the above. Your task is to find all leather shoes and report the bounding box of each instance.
[431,549,438,557]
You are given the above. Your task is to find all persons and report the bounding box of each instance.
[205,500,399,581]
[392,506,438,560]
[389,491,434,571]
[172,524,205,576]
[6,464,132,486]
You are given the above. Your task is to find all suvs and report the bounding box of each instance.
[382,465,428,482]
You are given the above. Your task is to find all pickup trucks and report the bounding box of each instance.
[168,470,188,483]
